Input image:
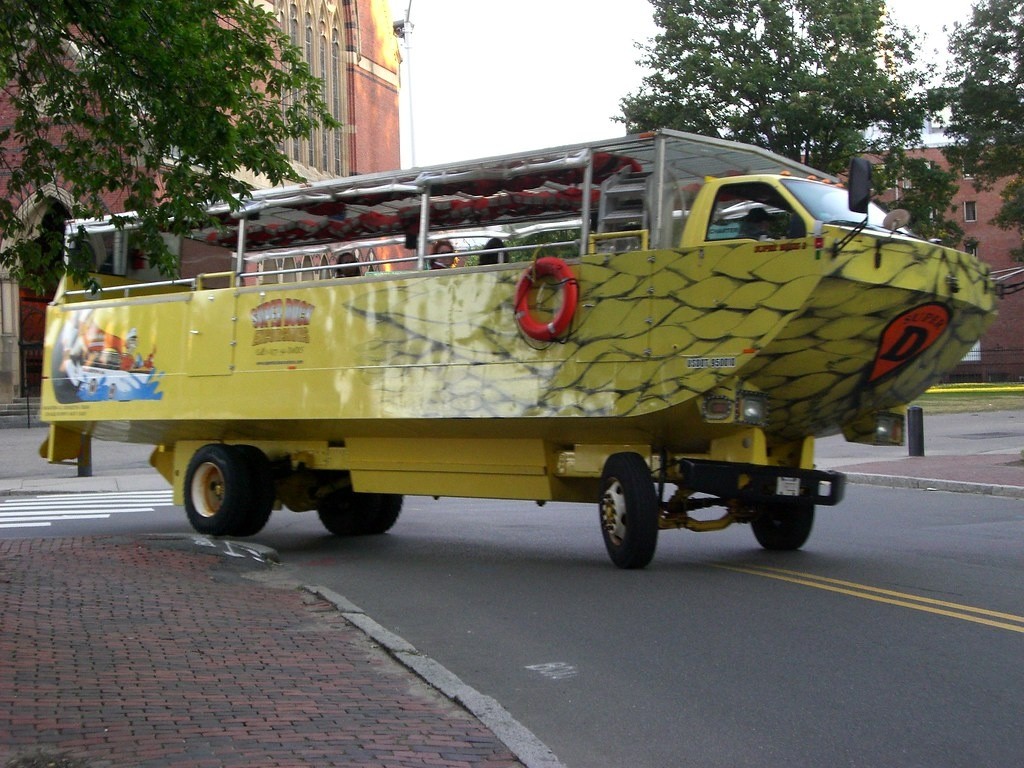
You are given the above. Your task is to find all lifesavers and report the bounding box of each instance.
[515,259,577,338]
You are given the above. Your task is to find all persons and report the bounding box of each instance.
[478,237,510,265]
[428,240,456,269]
[335,252,361,278]
